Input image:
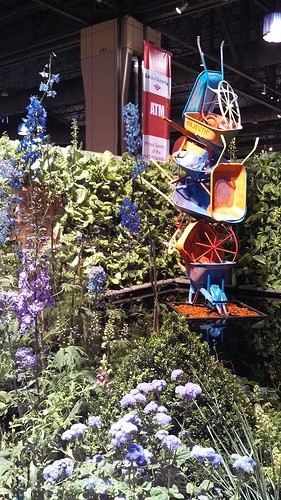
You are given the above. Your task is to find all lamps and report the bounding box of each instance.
[176,1,189,14]
[262,11,281,43]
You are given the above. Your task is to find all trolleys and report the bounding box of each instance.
[138,35,262,316]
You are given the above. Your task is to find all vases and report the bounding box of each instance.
[166,299,267,334]
